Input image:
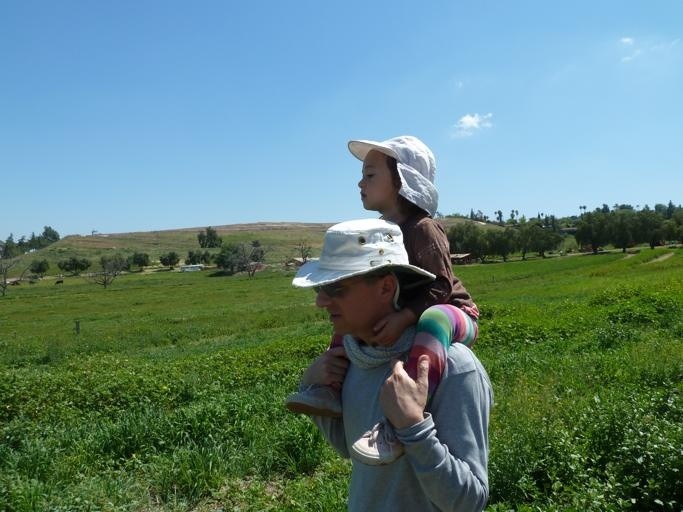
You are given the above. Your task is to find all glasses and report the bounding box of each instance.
[314,274,374,297]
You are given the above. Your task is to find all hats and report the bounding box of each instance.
[292,218,436,288]
[348,136,438,218]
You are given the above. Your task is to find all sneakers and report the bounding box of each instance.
[351,417,405,464]
[286,384,342,417]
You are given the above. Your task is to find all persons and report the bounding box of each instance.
[297,217,496,512]
[285,135,479,467]
